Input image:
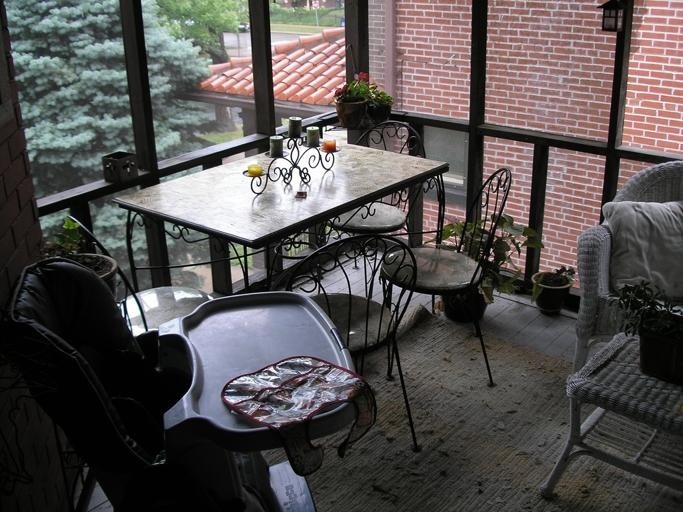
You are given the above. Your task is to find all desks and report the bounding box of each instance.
[111,139,450,294]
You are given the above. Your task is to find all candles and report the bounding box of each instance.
[322,137,336,152]
[248,164,263,176]
[307,126,319,147]
[270,135,284,157]
[288,116,301,139]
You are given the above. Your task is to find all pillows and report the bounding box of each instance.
[602,199,682,299]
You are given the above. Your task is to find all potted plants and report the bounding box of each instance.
[607,276,682,383]
[428,212,573,325]
[366,90,391,125]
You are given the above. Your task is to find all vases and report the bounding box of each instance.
[335,98,367,127]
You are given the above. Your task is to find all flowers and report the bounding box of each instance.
[334,72,376,101]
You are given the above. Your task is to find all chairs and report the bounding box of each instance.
[318,122,426,294]
[380,167,512,388]
[541,161,682,509]
[67,215,214,337]
[271,234,422,449]
[5,258,366,510]
